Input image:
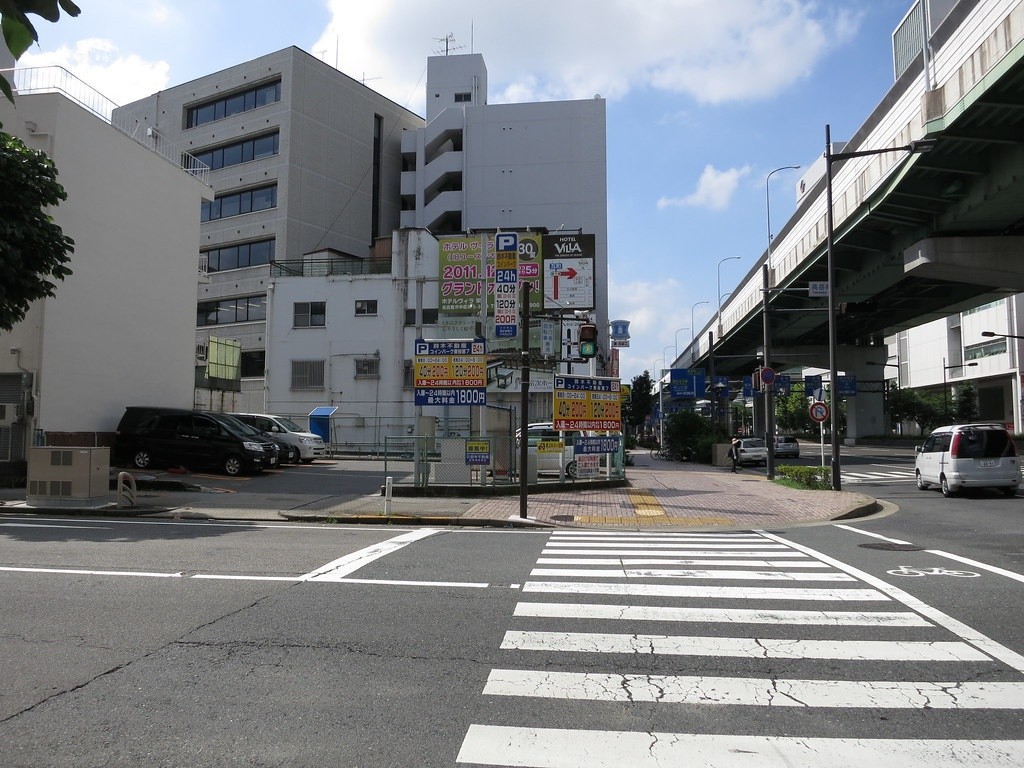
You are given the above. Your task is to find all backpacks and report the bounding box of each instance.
[728,446,734,457]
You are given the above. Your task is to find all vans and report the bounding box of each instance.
[914,423,1022,496]
[225,411,327,465]
[110,405,280,476]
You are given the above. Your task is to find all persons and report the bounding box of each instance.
[730,438,739,474]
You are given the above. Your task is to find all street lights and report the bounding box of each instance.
[943,357,979,423]
[691,301,710,365]
[866,356,903,439]
[716,255,742,339]
[823,123,939,498]
[763,165,801,480]
[663,345,675,381]
[675,327,689,367]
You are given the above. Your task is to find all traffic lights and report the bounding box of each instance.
[578,322,598,358]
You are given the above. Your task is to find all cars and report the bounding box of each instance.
[732,438,769,467]
[246,425,297,466]
[471,421,595,478]
[773,435,800,459]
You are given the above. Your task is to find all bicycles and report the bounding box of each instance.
[650,445,683,463]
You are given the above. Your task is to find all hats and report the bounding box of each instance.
[732,438,738,444]
